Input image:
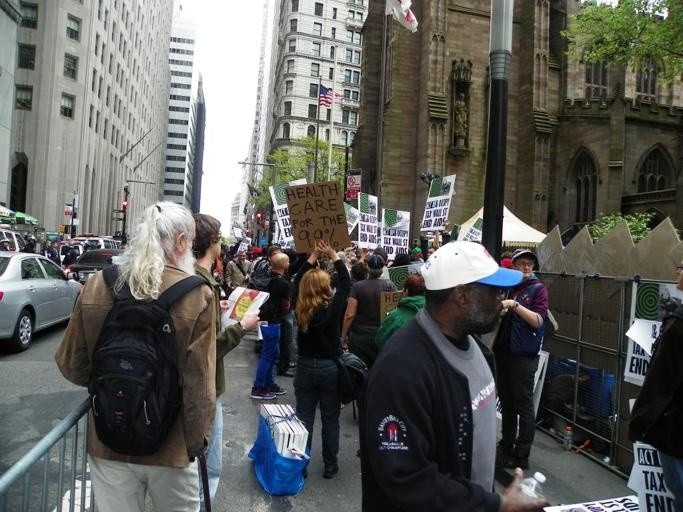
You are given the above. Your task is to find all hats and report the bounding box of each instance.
[510,247,540,271]
[419,240,524,292]
[367,254,384,270]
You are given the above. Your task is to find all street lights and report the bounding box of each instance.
[239,160,277,250]
[481,1,515,270]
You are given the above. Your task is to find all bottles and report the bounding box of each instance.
[562,426,574,453]
[519,470,547,501]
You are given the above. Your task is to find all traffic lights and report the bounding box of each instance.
[256,210,264,221]
[123,201,128,210]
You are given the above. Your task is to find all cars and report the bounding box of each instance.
[1,222,129,355]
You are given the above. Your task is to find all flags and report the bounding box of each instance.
[319,83,344,108]
[385,0,419,33]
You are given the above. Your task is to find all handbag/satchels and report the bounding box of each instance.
[335,347,370,421]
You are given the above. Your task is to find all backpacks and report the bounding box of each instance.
[247,273,282,320]
[545,308,559,334]
[85,262,210,459]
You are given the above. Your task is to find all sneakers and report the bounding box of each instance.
[248,386,277,400]
[494,441,530,473]
[271,386,288,396]
[275,372,295,378]
[322,463,338,479]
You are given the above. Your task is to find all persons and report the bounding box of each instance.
[0,234,77,269]
[54,197,218,512]
[627,256,682,512]
